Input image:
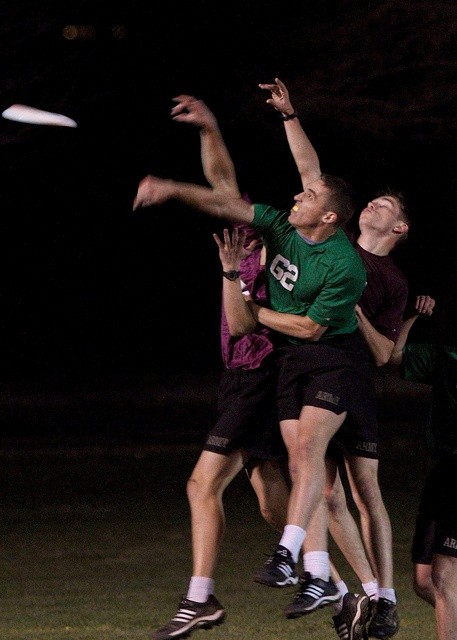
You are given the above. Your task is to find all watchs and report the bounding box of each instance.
[220,269,243,281]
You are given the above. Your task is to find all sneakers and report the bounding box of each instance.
[284,572,342,619]
[368,590,400,640]
[153,594,225,640]
[332,592,369,640]
[253,544,299,587]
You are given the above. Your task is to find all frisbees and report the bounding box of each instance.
[2,104,76,128]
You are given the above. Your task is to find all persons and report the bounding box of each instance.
[149,90,368,639]
[390,295,456,640]
[257,75,410,640]
[132,171,369,619]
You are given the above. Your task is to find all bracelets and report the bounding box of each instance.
[277,107,303,121]
